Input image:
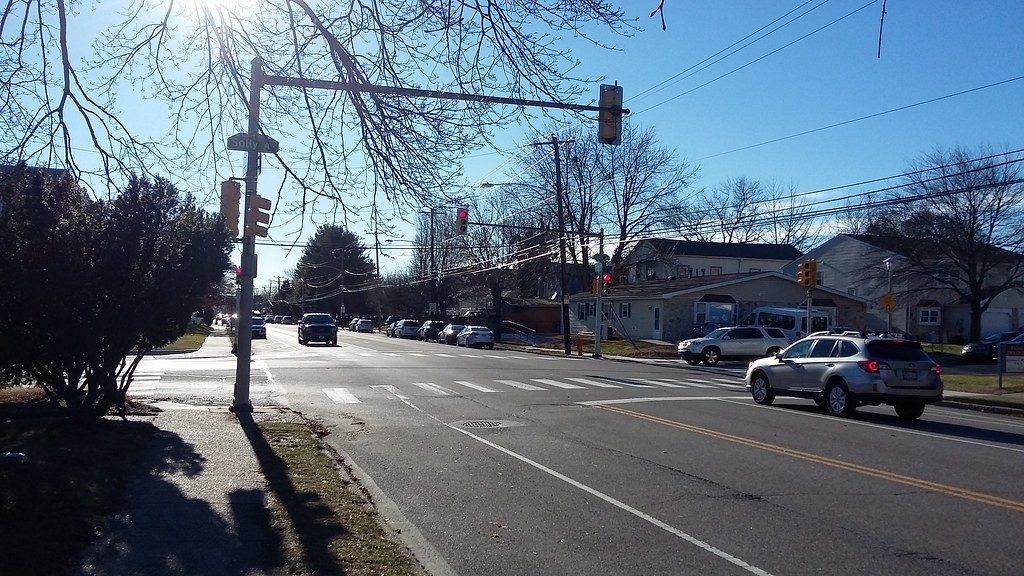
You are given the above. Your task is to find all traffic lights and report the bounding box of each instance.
[602,273,611,296]
[802,260,815,287]
[456,209,469,235]
[246,192,271,238]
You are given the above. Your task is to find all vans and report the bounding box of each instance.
[737,307,830,344]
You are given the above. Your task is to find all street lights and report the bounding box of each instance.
[481,137,576,354]
[883,257,898,335]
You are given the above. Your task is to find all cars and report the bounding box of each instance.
[417,320,446,343]
[355,319,373,333]
[386,321,399,337]
[438,323,467,345]
[393,319,421,339]
[992,333,1024,363]
[297,312,338,345]
[960,331,1022,364]
[386,315,402,325]
[215,309,293,339]
[456,325,496,350]
[349,318,360,332]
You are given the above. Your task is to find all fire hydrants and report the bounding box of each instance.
[575,335,583,356]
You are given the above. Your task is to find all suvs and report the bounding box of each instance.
[677,326,789,367]
[745,330,945,420]
